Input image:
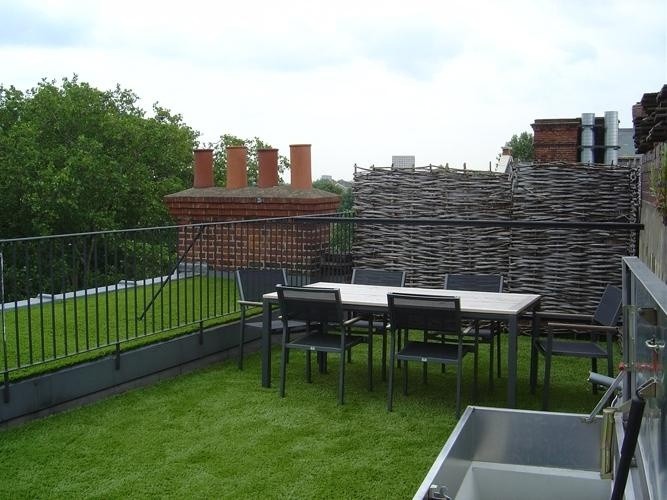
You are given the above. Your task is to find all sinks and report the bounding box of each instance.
[412,463,637,500]
[413,405,635,476]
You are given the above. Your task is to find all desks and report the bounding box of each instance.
[262,281,542,410]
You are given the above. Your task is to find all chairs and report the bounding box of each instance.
[275,283,373,404]
[386,292,479,420]
[423,272,504,388]
[528,283,623,410]
[234,268,327,388]
[313,267,407,380]
